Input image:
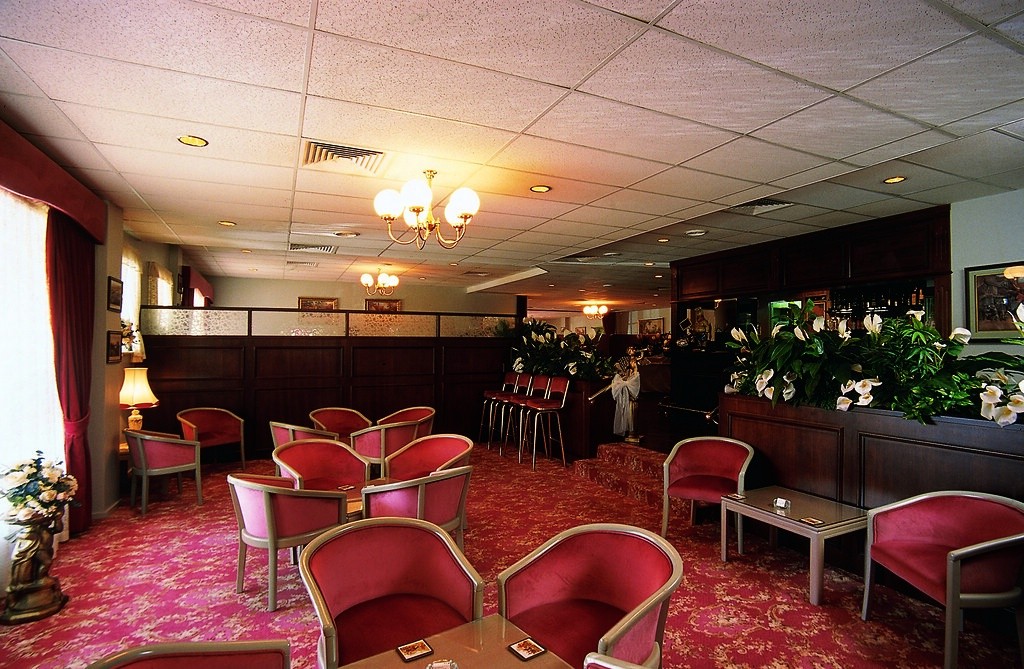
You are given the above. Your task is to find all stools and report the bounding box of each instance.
[481,372,570,471]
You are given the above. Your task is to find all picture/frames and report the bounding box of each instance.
[177,273,183,294]
[575,327,586,335]
[638,317,664,334]
[364,298,401,311]
[107,276,124,313]
[297,296,338,309]
[106,330,122,364]
[963,260,1024,345]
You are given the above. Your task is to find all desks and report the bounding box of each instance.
[637,349,726,394]
[721,484,868,606]
[337,612,573,669]
[327,477,405,523]
[338,433,350,448]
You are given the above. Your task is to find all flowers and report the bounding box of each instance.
[509,297,1024,428]
[121,317,137,351]
[0,449,79,520]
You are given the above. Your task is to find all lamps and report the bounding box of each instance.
[583,305,608,320]
[373,169,480,250]
[1003,266,1024,289]
[119,368,160,430]
[360,268,400,296]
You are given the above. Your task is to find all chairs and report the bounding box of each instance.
[861,490,1024,669]
[272,439,372,564]
[375,407,435,439]
[122,427,203,518]
[85,639,291,669]
[227,472,346,613]
[309,408,372,435]
[660,436,754,555]
[384,434,474,532]
[269,421,339,475]
[361,465,474,557]
[350,420,423,465]
[497,523,684,669]
[299,517,486,669]
[176,407,246,472]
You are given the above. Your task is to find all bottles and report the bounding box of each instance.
[825,286,925,330]
[133,325,146,362]
